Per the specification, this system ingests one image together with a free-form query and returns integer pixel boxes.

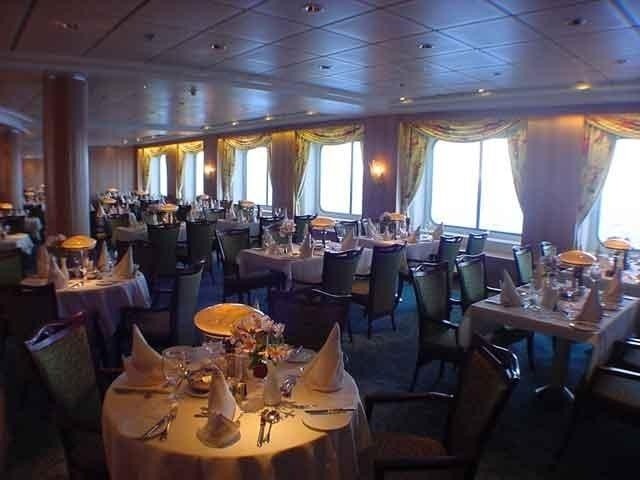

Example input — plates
[566,319,603,333]
[112,374,177,393]
[286,348,317,363]
[301,407,353,430]
[181,386,212,399]
[117,412,167,439]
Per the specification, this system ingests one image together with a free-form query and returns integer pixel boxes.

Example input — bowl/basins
[187,369,217,391]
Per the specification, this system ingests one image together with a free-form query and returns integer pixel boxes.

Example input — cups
[230,381,246,399]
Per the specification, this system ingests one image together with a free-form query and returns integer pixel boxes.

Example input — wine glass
[162,349,186,402]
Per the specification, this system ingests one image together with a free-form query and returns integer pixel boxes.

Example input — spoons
[263,410,281,444]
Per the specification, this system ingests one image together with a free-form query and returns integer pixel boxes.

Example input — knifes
[256,408,268,448]
[304,408,357,415]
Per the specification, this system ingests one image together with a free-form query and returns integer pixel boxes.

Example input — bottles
[263,360,281,406]
